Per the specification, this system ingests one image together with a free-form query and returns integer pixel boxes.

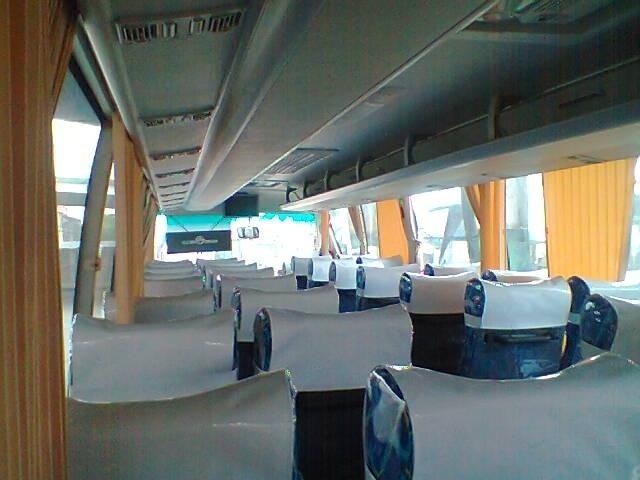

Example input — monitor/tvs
[225,193,259,217]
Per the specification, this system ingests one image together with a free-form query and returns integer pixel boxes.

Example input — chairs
[67,366,298,477]
[464,274,572,377]
[573,292,639,367]
[362,351,638,475]
[100,252,637,321]
[252,304,415,475]
[66,306,239,405]
